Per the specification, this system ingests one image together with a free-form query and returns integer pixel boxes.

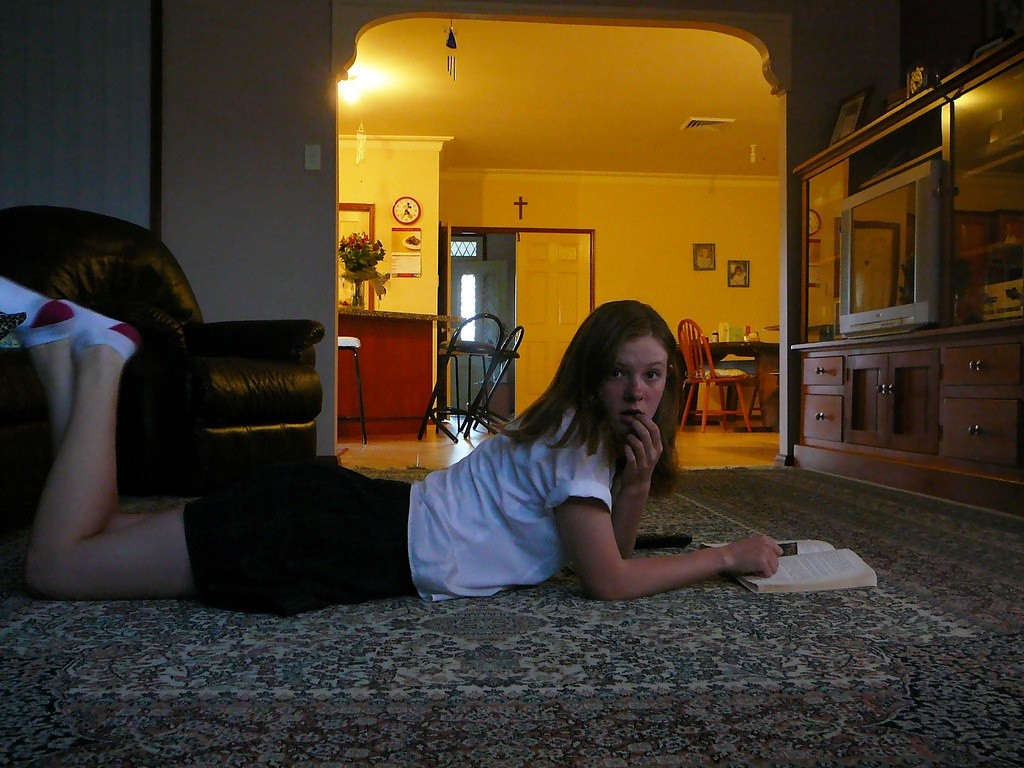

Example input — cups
[819,325,833,340]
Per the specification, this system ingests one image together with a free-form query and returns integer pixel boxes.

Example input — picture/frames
[827,85,871,147]
[728,260,749,287]
[692,243,716,271]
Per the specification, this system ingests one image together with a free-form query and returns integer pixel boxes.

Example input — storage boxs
[728,327,744,341]
[951,278,1024,326]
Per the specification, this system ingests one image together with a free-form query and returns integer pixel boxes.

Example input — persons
[1,275,786,611]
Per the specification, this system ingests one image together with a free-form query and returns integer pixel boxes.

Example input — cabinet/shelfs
[789,316,1024,470]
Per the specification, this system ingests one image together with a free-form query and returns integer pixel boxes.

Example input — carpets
[0,466,1024,768]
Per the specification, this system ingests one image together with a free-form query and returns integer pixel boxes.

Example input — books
[700,538,878,594]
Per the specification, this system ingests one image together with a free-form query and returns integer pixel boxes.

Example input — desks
[662,341,780,431]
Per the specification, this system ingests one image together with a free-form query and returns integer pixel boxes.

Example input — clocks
[809,209,822,236]
[392,196,421,224]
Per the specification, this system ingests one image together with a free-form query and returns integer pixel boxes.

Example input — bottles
[701,331,718,344]
[744,326,760,342]
[718,319,729,342]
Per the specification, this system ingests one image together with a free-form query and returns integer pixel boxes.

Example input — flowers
[339,231,389,311]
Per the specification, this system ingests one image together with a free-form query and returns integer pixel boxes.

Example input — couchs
[0,205,324,517]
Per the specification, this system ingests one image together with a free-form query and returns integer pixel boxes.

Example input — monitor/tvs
[839,158,945,339]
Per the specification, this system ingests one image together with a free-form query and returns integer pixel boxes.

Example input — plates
[402,238,420,250]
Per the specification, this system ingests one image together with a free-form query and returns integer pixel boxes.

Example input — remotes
[634,533,693,551]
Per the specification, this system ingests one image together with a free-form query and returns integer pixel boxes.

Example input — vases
[351,278,365,307]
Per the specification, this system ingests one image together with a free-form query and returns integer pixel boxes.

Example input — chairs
[677,320,754,433]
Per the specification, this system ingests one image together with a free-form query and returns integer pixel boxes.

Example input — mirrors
[338,203,375,311]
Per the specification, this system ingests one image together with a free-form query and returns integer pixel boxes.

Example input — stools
[416,313,525,445]
[338,336,368,445]
